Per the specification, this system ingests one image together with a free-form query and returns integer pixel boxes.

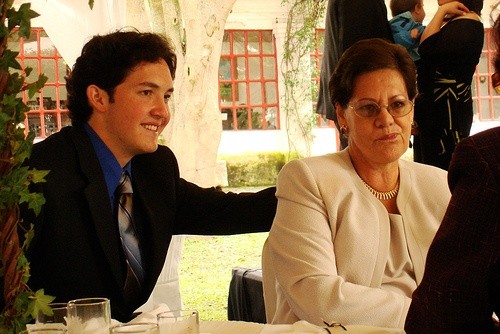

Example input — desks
[25,319,406,334]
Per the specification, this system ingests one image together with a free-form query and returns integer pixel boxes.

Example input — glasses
[341,99,418,118]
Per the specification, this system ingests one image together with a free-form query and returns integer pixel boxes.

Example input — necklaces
[361,178,400,200]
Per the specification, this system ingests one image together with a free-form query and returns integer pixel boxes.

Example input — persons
[16,26,278,322]
[404,14,500,334]
[316,0,396,151]
[388,0,484,171]
[268,39,452,329]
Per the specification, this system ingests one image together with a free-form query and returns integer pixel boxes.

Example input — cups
[109,322,160,334]
[156,309,200,334]
[67,298,111,334]
[35,302,68,334]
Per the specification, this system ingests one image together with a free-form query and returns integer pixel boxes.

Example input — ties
[116,173,144,310]
